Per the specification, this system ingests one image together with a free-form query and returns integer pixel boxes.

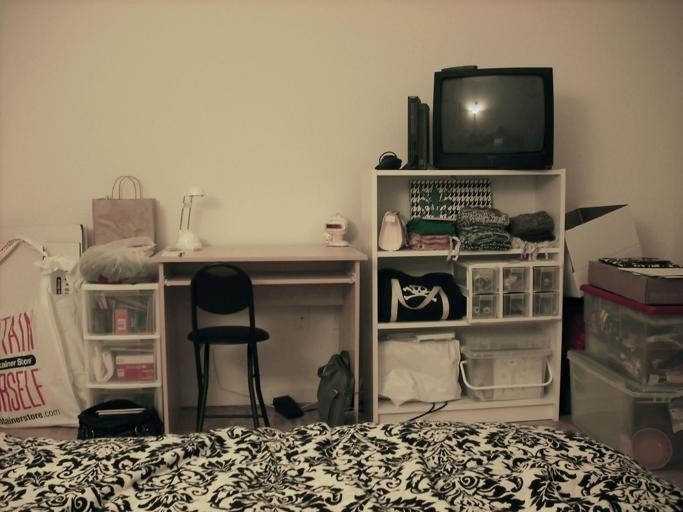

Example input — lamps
[174,187,206,251]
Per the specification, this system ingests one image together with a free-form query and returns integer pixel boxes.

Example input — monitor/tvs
[433,67,554,169]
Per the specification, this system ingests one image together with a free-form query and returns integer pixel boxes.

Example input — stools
[184,262,272,430]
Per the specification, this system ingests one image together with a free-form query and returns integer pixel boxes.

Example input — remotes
[441,65,477,72]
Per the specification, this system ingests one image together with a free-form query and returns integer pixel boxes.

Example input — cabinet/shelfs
[369,164,568,429]
[81,276,163,425]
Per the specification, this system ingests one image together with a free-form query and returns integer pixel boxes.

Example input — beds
[0,419,681,512]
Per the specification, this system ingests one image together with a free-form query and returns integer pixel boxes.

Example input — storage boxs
[457,324,552,351]
[566,346,683,469]
[564,204,644,300]
[451,259,561,319]
[580,281,683,387]
[459,344,555,400]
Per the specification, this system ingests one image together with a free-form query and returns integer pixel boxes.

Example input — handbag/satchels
[379,210,407,251]
[378,270,466,323]
[77,398,163,438]
[315,351,354,426]
[92,176,155,247]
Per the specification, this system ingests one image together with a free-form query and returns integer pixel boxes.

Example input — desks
[150,241,369,432]
